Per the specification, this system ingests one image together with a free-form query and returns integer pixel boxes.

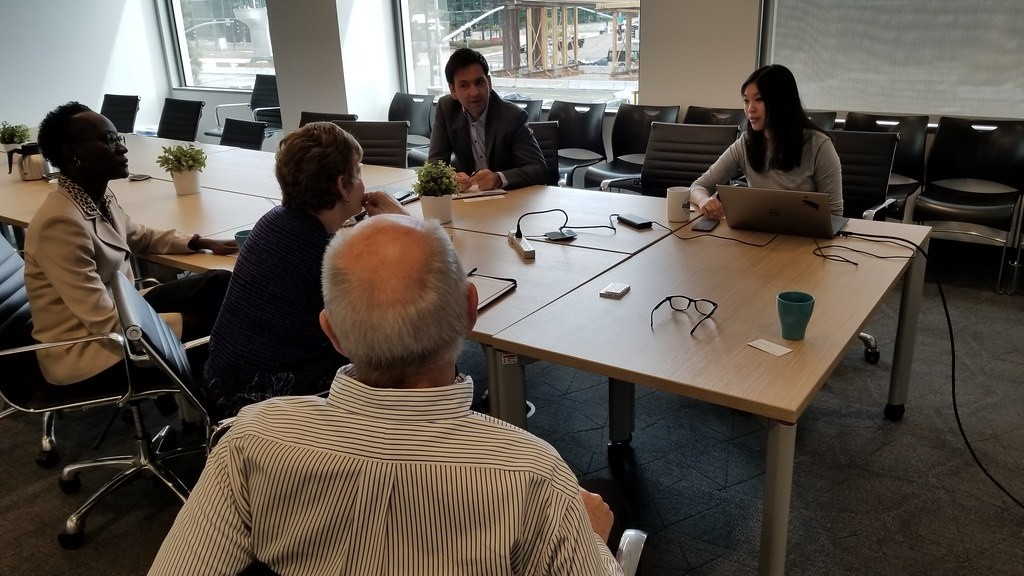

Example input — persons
[428,47,548,191]
[688,64,843,221]
[203,122,411,441]
[24,102,238,395]
[152,215,625,576]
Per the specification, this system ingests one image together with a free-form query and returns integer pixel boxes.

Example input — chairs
[100,75,1024,296]
[0,233,208,552]
[110,270,331,454]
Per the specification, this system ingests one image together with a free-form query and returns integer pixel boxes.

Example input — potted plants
[0,121,33,164]
[413,159,462,224]
[156,143,207,196]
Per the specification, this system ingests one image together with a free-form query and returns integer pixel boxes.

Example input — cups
[667,186,691,223]
[776,291,814,341]
[234,229,252,250]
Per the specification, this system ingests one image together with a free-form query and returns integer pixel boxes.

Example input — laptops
[716,184,850,239]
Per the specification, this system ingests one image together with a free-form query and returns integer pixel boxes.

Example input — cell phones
[692,217,720,232]
[393,191,410,200]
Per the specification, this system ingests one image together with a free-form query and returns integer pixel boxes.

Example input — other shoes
[180,428,204,447]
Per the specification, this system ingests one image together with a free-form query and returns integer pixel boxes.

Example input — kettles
[7,142,51,180]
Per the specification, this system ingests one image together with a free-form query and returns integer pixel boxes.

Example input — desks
[0,125,933,576]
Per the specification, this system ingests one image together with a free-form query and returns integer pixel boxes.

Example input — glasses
[651,295,717,335]
[80,132,125,149]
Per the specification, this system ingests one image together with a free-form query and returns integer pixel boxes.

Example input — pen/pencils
[468,167,482,179]
[466,267,478,277]
[397,193,410,201]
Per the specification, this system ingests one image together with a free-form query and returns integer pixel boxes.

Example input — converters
[616,212,652,229]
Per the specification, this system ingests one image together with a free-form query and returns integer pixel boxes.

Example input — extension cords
[508,230,535,258]
